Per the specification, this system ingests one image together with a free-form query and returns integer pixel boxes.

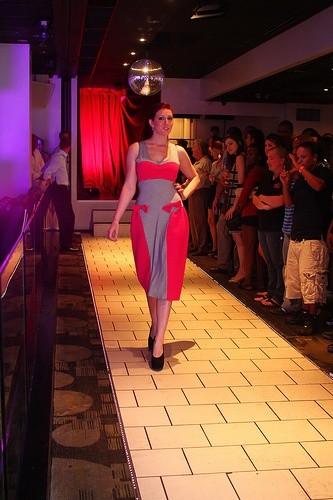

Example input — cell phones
[284,156,292,171]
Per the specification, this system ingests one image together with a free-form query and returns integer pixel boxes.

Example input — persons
[43,139,80,251]
[279,141,330,326]
[182,137,213,255]
[108,102,200,371]
[208,140,225,256]
[253,146,285,307]
[210,151,239,272]
[32,133,45,182]
[239,143,269,289]
[245,130,266,172]
[276,119,296,154]
[51,131,70,175]
[224,135,246,284]
[175,120,333,352]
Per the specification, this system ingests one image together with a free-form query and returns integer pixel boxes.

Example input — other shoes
[260,297,282,307]
[321,328,333,339]
[190,250,268,295]
[287,311,309,324]
[147,335,156,351]
[327,344,333,352]
[63,247,79,251]
[150,350,164,371]
[301,314,317,336]
[252,296,266,302]
[269,307,290,315]
